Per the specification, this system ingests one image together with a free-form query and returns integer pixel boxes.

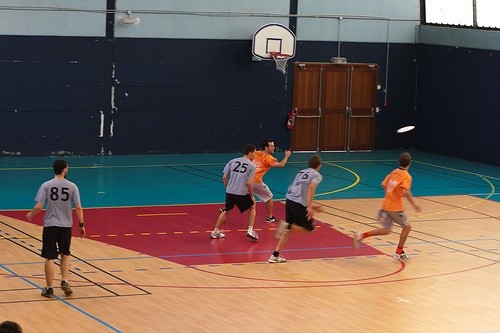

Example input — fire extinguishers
[286,106,298,129]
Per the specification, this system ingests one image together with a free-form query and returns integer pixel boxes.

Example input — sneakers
[41,287,53,298]
[61,280,73,296]
[268,254,286,262]
[266,216,279,222]
[210,231,225,239]
[393,252,410,261]
[247,230,258,239]
[354,232,362,248]
[276,221,287,238]
[220,208,225,213]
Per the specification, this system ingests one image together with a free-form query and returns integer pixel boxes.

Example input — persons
[210,145,259,240]
[268,156,322,263]
[26,160,85,298]
[0,320,22,333]
[352,151,420,261]
[250,140,291,223]
